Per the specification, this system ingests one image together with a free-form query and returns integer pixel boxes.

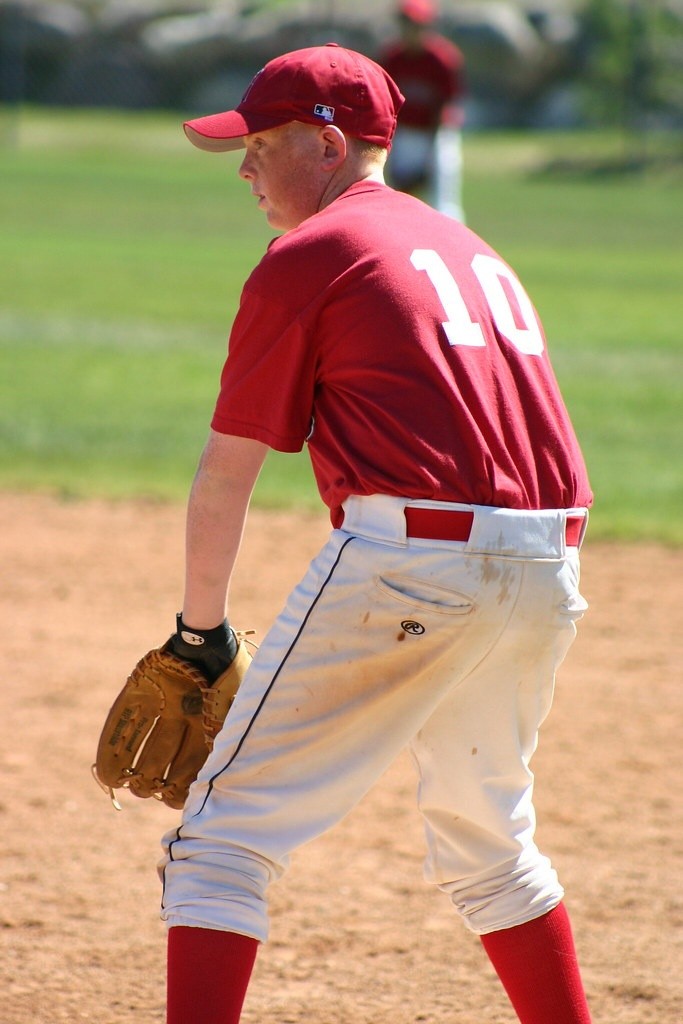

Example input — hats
[182,42,406,154]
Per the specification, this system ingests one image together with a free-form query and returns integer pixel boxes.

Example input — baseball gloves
[92,616,257,812]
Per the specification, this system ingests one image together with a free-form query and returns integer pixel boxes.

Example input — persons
[92,43,591,1024]
[368,0,467,220]
